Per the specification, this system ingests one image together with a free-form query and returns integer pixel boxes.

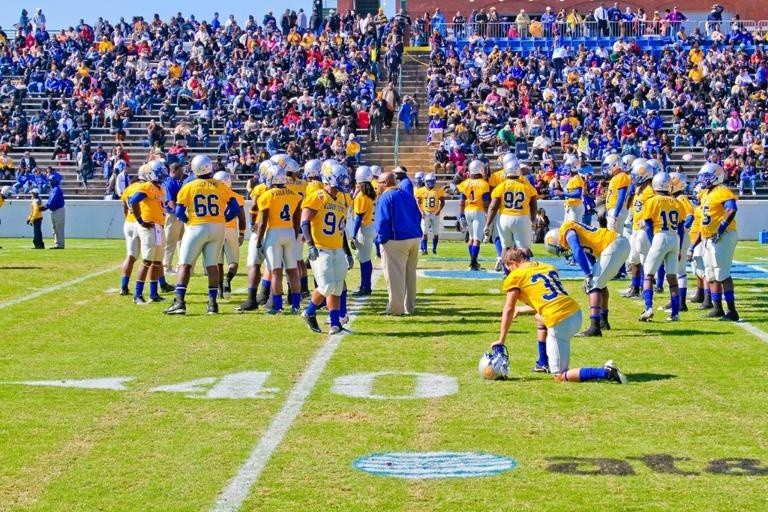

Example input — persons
[489,245,629,385]
[0,0,422,202]
[395,0,768,201]
[24,187,46,250]
[0,184,15,249]
[115,149,742,330]
[38,171,68,250]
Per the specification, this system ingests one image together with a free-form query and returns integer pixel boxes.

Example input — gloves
[612,216,617,228]
[459,217,467,228]
[712,231,721,244]
[239,224,357,270]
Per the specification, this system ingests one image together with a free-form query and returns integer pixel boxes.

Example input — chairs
[451,32,665,60]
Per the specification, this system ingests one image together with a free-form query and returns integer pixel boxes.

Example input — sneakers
[534,360,550,373]
[605,360,629,384]
[574,322,610,337]
[465,259,739,322]
[121,280,371,335]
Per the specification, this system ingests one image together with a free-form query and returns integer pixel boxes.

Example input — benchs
[661,108,708,180]
[8,91,247,199]
